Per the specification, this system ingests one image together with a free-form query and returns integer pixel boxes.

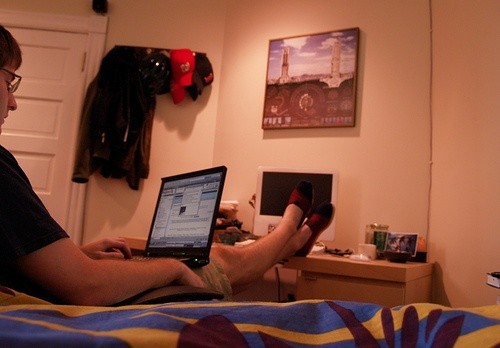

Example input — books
[486,272,500,289]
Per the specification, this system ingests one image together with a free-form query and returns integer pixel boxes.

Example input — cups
[358,244,376,260]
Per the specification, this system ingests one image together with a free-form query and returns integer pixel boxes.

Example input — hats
[169,48,195,86]
[187,53,214,95]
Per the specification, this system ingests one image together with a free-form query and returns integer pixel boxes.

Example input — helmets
[138,53,170,90]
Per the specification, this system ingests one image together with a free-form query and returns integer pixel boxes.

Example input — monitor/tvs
[253,169,338,242]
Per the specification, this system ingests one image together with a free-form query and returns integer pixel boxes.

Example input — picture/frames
[384,232,419,256]
[261,26,360,130]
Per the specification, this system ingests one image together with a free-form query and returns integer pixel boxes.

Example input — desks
[120,236,435,308]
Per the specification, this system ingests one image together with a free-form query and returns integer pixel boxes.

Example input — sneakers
[282,181,314,230]
[294,201,335,256]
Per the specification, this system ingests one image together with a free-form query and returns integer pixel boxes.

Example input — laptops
[129,165,228,270]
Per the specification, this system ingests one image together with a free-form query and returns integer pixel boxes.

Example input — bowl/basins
[384,251,411,263]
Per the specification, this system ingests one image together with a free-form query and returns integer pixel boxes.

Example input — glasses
[0,68,22,94]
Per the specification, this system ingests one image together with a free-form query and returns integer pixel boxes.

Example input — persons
[0,25,336,307]
